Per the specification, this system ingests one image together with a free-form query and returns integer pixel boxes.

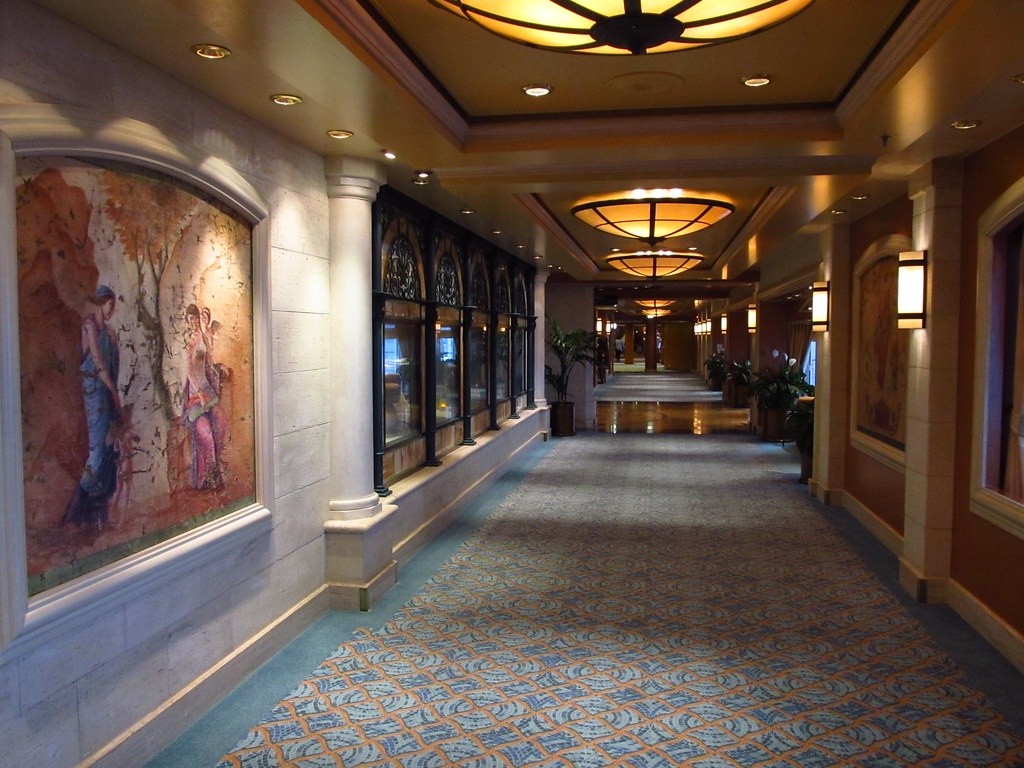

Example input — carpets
[144,433,1024,768]
[594,373,725,402]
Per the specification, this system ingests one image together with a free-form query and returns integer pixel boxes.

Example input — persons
[656,333,661,362]
[597,337,610,384]
[615,336,624,362]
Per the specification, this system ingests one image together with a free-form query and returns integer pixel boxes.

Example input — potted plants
[704,357,724,391]
[751,358,815,442]
[545,315,608,438]
[725,360,753,408]
[598,337,609,384]
[785,398,814,484]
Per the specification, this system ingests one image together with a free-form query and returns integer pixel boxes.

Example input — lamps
[435,320,441,334]
[721,313,727,334]
[634,300,677,308]
[694,323,698,335]
[426,0,816,56]
[812,282,828,332]
[897,251,924,329]
[571,197,736,247]
[707,319,712,335]
[641,309,671,318]
[698,322,702,335]
[605,321,610,335]
[605,254,704,278]
[702,320,706,335]
[610,322,617,330]
[596,318,602,334]
[748,304,756,333]
